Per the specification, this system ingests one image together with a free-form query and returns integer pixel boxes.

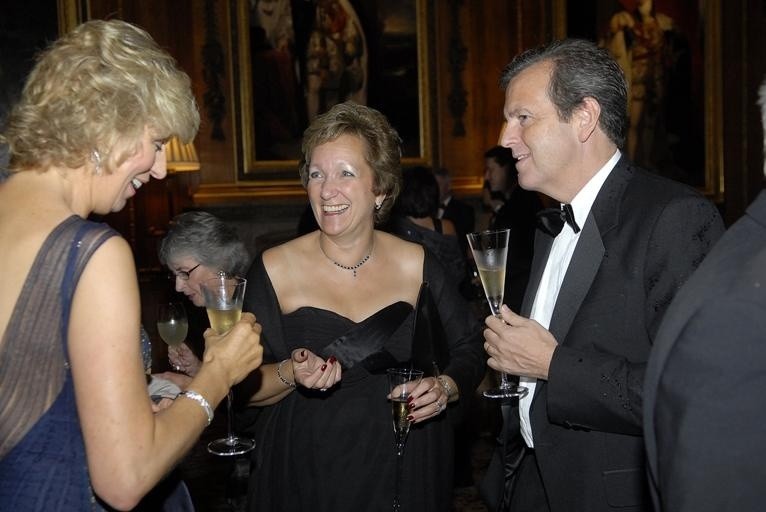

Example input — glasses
[167,264,200,280]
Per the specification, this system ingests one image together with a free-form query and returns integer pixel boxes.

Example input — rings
[435,400,441,409]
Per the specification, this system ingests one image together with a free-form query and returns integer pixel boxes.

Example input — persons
[0,18,265,512]
[143,209,257,393]
[401,146,545,315]
[235,96,477,512]
[480,36,726,512]
[640,78,765,512]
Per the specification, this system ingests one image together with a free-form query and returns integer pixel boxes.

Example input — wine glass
[154,298,190,377]
[464,227,530,398]
[197,275,257,457]
[382,366,426,511]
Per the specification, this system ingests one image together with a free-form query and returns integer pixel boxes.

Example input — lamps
[162,133,201,225]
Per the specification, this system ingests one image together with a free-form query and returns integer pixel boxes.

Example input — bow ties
[535,204,580,237]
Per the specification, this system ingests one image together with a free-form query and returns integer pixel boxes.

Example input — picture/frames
[226,1,439,190]
[0,0,95,180]
[551,1,727,207]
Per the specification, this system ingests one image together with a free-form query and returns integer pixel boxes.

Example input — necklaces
[321,232,373,280]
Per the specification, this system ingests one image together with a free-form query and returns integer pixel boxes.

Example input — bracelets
[276,358,297,389]
[182,391,214,423]
[434,376,453,400]
